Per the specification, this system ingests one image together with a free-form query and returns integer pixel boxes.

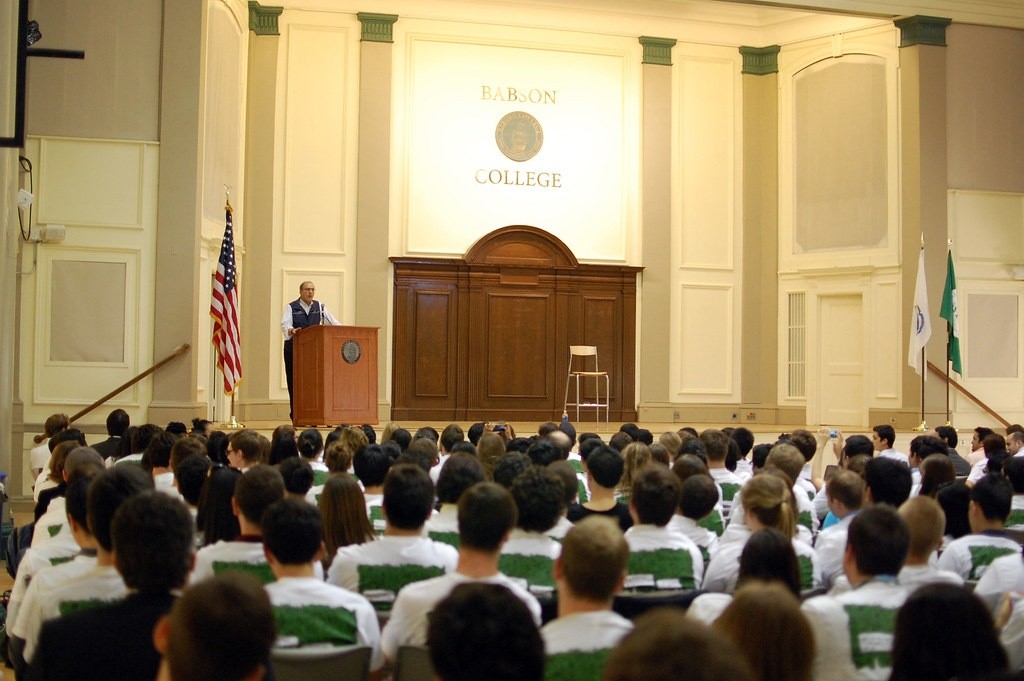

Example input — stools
[563,345,610,433]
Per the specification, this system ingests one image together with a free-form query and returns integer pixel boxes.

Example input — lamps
[39,224,66,244]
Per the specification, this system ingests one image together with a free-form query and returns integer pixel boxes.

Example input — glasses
[225,449,236,456]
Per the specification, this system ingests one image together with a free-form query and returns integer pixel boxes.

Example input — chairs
[393,644,434,681]
[271,645,372,681]
[613,588,709,621]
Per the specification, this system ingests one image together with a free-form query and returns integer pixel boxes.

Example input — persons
[6,409,1024,681]
[281,281,342,422]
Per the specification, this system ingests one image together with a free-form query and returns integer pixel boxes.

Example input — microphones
[321,304,325,317]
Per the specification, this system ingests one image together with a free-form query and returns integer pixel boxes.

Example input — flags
[210,202,244,396]
[939,250,963,377]
[907,242,931,381]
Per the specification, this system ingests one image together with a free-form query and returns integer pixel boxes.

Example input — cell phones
[494,424,507,432]
[830,430,839,438]
